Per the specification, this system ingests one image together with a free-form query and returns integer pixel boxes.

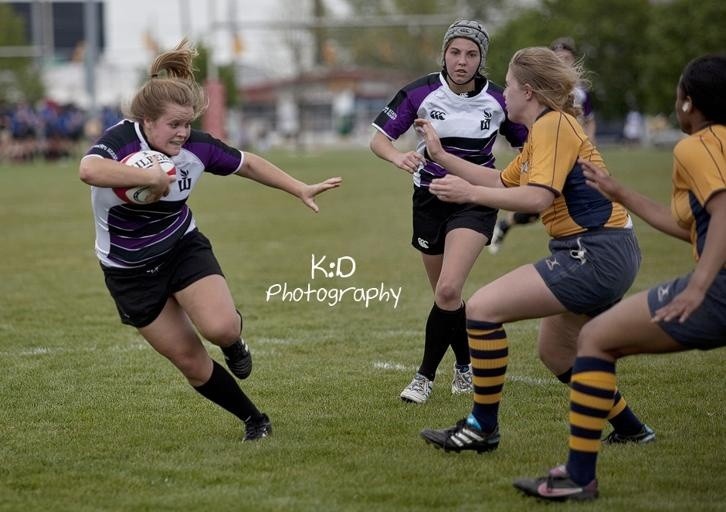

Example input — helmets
[442,17,491,63]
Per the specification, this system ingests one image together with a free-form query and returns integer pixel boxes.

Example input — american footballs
[111,151,176,204]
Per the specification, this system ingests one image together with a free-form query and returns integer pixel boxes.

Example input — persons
[1,94,129,166]
[367,16,530,406]
[77,35,344,444]
[411,44,658,455]
[509,45,726,503]
[484,35,600,261]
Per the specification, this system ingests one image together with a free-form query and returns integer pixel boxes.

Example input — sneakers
[221,337,252,380]
[450,360,472,395]
[601,424,656,446]
[399,373,434,404]
[241,412,272,443]
[512,464,599,502]
[420,418,500,455]
